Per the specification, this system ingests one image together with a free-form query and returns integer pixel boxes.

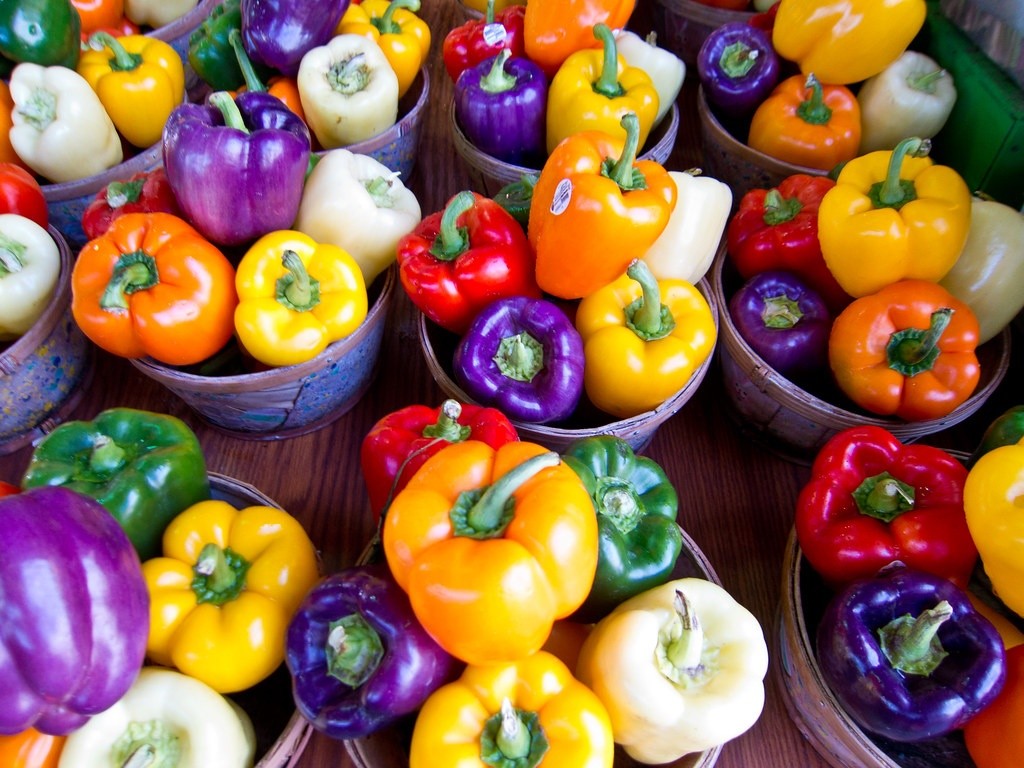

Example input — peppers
[1,1,1023,766]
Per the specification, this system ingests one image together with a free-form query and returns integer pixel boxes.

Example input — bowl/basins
[206,471,316,768]
[774,448,1024,768]
[143,1,223,100]
[1,222,90,457]
[130,260,396,441]
[706,235,1012,469]
[461,1,484,22]
[344,523,727,768]
[40,139,166,249]
[417,278,719,457]
[660,0,757,68]
[449,94,679,199]
[312,64,431,189]
[697,84,830,213]
